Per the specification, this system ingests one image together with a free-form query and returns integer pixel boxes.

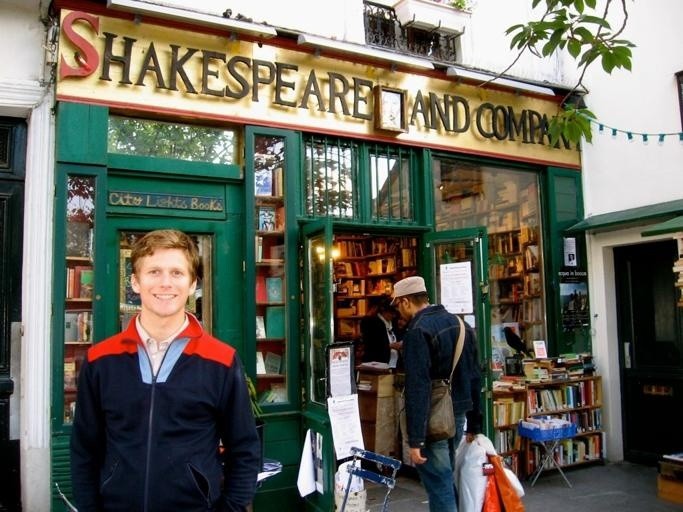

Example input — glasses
[387,328,395,342]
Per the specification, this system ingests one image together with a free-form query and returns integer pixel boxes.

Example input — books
[249,150,296,405]
[57,219,91,432]
[331,236,419,371]
[488,351,603,474]
[486,227,545,351]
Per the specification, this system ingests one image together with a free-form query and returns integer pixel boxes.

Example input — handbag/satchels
[451,433,526,512]
[398,378,457,447]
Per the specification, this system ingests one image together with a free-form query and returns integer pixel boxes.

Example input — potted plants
[391,0,472,34]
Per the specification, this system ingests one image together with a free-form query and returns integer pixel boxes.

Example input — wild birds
[502,326,534,360]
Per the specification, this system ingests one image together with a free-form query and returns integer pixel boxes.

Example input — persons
[67,229,262,512]
[387,274,485,511]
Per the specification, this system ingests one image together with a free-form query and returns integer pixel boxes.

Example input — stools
[527,435,575,489]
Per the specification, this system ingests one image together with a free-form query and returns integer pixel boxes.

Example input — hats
[389,275,427,307]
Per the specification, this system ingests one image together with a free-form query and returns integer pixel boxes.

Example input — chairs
[339,445,402,511]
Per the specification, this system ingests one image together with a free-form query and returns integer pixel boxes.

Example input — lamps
[295,30,435,79]
[446,68,555,98]
[105,0,277,39]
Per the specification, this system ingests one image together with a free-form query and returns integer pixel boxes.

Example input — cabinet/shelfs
[63,256,94,406]
[489,372,604,481]
[433,182,544,365]
[300,233,421,365]
[256,164,287,403]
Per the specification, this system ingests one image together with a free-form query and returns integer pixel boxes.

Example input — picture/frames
[117,248,197,315]
[374,85,410,135]
